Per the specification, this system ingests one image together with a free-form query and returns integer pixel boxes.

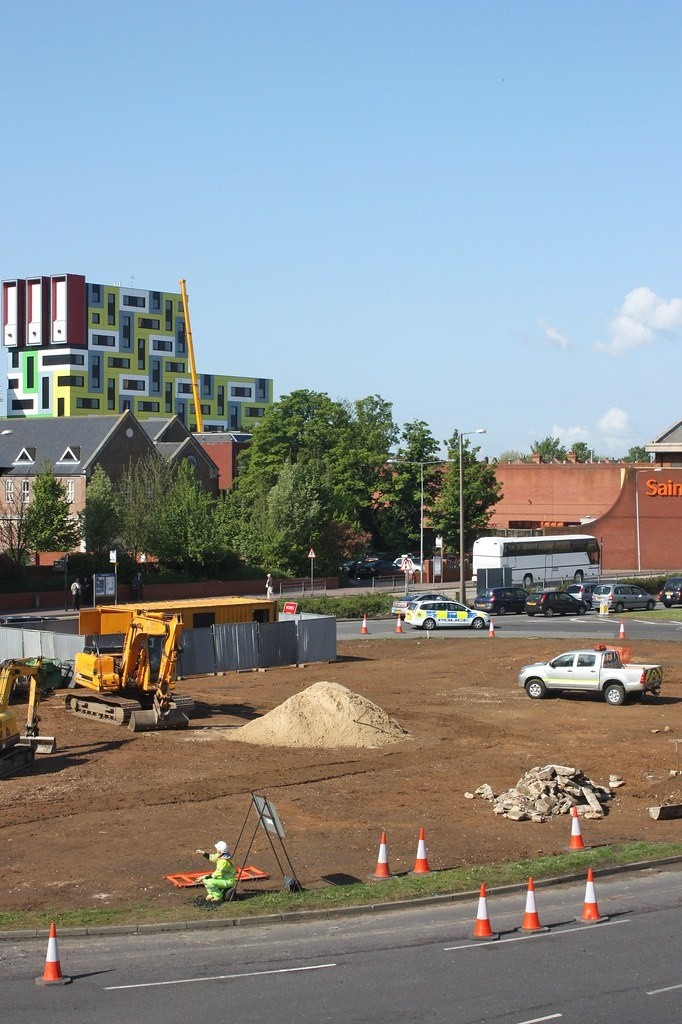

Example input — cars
[658,578,682,608]
[337,552,459,578]
[565,583,656,613]
[474,587,589,617]
[391,594,491,630]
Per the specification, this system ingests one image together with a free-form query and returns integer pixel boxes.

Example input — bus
[471,534,601,587]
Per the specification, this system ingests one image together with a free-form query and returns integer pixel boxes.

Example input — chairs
[580,655,595,665]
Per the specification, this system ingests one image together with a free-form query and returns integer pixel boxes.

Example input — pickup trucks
[518,649,663,706]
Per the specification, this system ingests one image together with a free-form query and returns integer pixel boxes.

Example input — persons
[265,574,274,599]
[196,841,236,903]
[131,572,144,602]
[71,576,91,611]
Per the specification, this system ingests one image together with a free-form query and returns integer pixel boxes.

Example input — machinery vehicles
[0,656,56,777]
[178,279,204,432]
[63,605,196,732]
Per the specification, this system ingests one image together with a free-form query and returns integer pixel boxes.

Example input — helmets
[215,841,229,853]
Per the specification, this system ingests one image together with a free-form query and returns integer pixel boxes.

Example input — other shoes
[211,898,223,902]
[205,895,214,900]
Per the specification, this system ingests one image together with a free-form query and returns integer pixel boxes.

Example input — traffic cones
[563,807,592,851]
[407,828,436,876]
[616,619,627,640]
[489,618,495,638]
[361,613,405,635]
[573,869,609,924]
[514,877,549,934]
[35,922,71,987]
[367,828,398,882]
[467,884,500,940]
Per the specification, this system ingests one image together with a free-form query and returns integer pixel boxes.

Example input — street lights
[636,468,662,572]
[387,429,486,604]
